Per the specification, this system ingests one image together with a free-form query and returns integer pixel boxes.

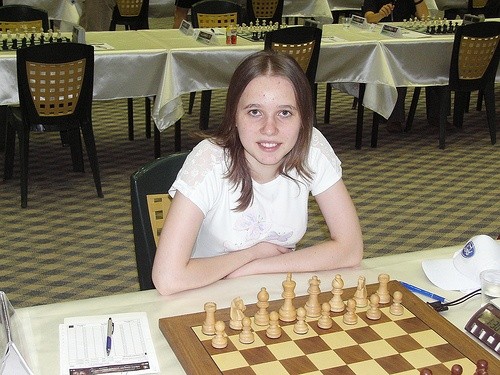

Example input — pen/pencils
[211,29,215,33]
[399,281,450,303]
[106,318,114,356]
[391,10,393,21]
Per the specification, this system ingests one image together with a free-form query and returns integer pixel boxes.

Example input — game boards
[398,25,456,36]
[235,26,288,41]
[157,279,500,375]
[0,36,72,55]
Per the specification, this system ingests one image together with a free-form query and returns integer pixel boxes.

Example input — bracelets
[414,0,424,5]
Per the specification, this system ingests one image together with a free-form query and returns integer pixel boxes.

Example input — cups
[341,18,351,29]
[480,268,500,309]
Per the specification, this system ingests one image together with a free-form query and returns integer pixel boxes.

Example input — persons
[360,0,456,132]
[152,50,363,296]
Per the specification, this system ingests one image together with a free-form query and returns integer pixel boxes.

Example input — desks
[136,24,378,152]
[326,18,500,148]
[0,239,500,375]
[0,30,169,161]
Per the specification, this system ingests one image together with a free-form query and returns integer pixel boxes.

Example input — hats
[421,235,500,292]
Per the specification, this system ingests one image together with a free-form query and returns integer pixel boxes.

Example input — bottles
[225,24,237,45]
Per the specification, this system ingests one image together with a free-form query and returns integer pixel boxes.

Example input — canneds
[225,25,237,45]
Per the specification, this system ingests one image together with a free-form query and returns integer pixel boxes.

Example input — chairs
[108,0,149,31]
[186,0,254,131]
[246,0,284,26]
[4,43,103,206]
[405,21,500,150]
[0,5,70,151]
[265,25,322,128]
